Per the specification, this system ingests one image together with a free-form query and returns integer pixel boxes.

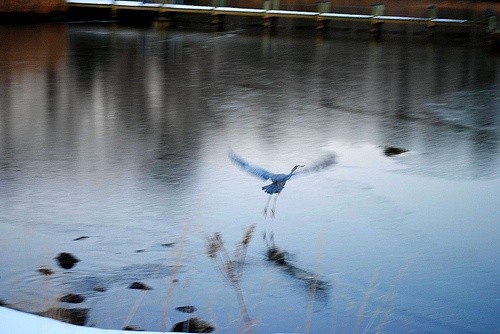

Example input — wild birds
[227,149,337,220]
